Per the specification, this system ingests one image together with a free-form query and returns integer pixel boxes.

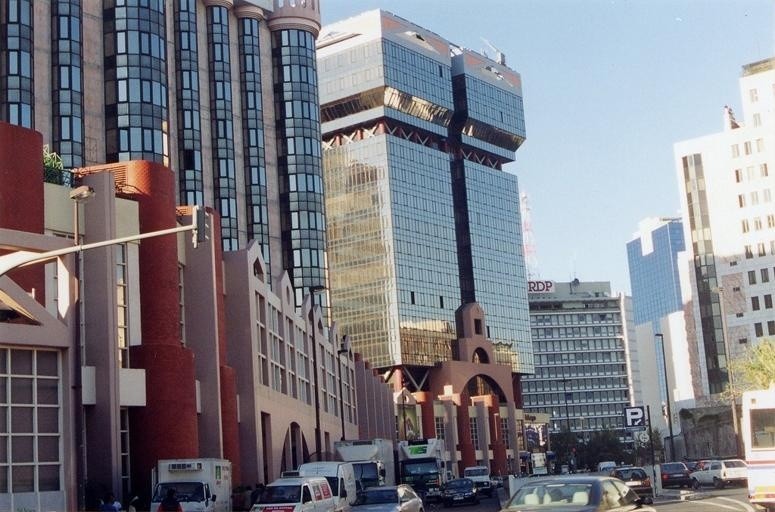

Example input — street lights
[655,333,678,464]
[557,378,574,435]
[711,285,743,461]
[307,281,333,463]
[338,348,348,443]
[67,184,97,510]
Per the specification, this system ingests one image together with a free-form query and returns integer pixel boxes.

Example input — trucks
[248,436,451,512]
[147,456,235,512]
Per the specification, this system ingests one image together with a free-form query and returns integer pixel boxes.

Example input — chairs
[572,492,589,504]
[755,430,773,447]
[523,494,539,505]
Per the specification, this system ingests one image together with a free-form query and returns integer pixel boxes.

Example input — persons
[233,483,264,510]
[95,488,184,512]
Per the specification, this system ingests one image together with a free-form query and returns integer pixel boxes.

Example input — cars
[490,474,505,487]
[502,476,665,512]
[440,474,482,506]
[557,457,754,507]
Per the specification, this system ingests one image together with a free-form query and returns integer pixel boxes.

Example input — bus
[740,390,775,512]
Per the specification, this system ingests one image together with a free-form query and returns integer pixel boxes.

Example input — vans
[463,466,497,497]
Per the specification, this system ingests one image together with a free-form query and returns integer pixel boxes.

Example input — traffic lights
[204,211,211,243]
